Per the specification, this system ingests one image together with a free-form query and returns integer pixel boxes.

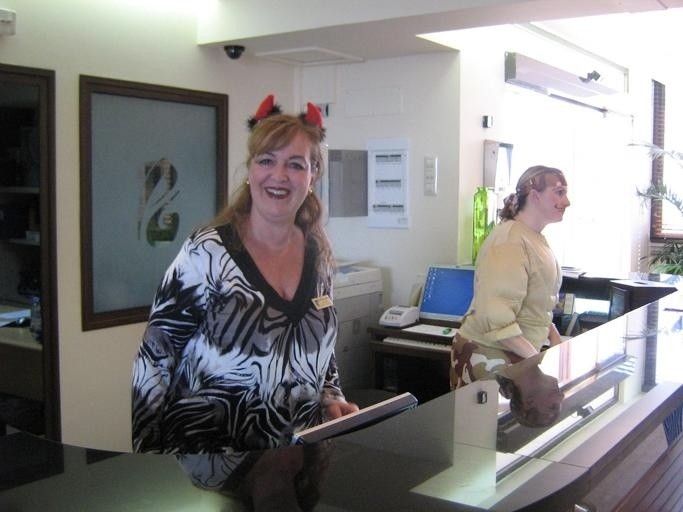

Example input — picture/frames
[78,71,229,331]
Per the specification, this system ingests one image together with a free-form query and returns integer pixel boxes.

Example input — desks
[365,317,461,390]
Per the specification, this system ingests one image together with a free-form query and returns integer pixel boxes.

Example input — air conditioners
[504,52,619,114]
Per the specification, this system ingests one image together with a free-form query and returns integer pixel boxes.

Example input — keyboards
[382,336,452,352]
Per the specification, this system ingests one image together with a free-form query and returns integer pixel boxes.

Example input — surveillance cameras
[224,45,245,60]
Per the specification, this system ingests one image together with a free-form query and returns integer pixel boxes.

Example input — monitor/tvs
[607,280,677,319]
[417,264,475,322]
[288,391,419,444]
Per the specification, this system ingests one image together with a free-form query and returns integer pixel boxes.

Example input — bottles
[30,297,42,335]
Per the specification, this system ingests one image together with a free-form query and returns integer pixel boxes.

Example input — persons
[174,441,335,512]
[497,352,565,429]
[132,116,360,452]
[450,167,572,391]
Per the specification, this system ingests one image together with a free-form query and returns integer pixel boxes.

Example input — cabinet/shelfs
[0,187,44,405]
